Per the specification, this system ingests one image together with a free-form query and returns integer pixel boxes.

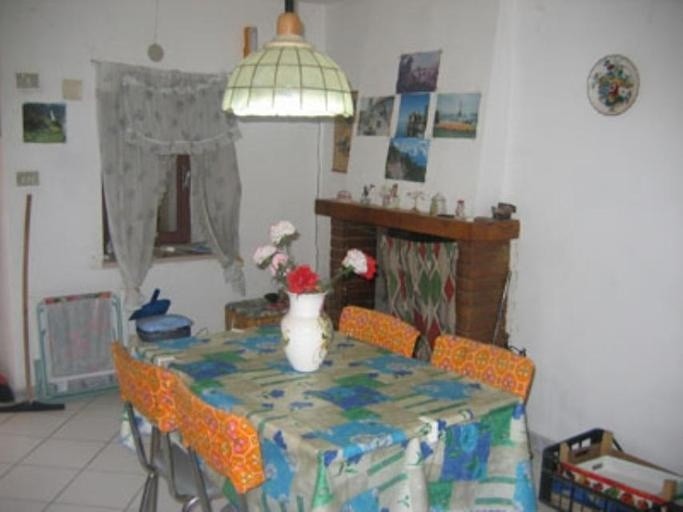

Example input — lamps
[217,0,361,122]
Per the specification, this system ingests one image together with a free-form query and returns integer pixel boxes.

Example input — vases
[276,283,334,376]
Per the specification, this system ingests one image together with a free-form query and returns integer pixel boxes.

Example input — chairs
[109,306,535,512]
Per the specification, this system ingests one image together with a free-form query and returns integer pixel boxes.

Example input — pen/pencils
[364,183,374,198]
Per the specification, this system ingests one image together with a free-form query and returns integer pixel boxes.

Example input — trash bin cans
[136,315,192,341]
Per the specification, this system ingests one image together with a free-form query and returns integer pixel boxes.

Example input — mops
[0,195,66,412]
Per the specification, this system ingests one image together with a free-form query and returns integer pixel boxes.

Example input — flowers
[250,218,375,301]
